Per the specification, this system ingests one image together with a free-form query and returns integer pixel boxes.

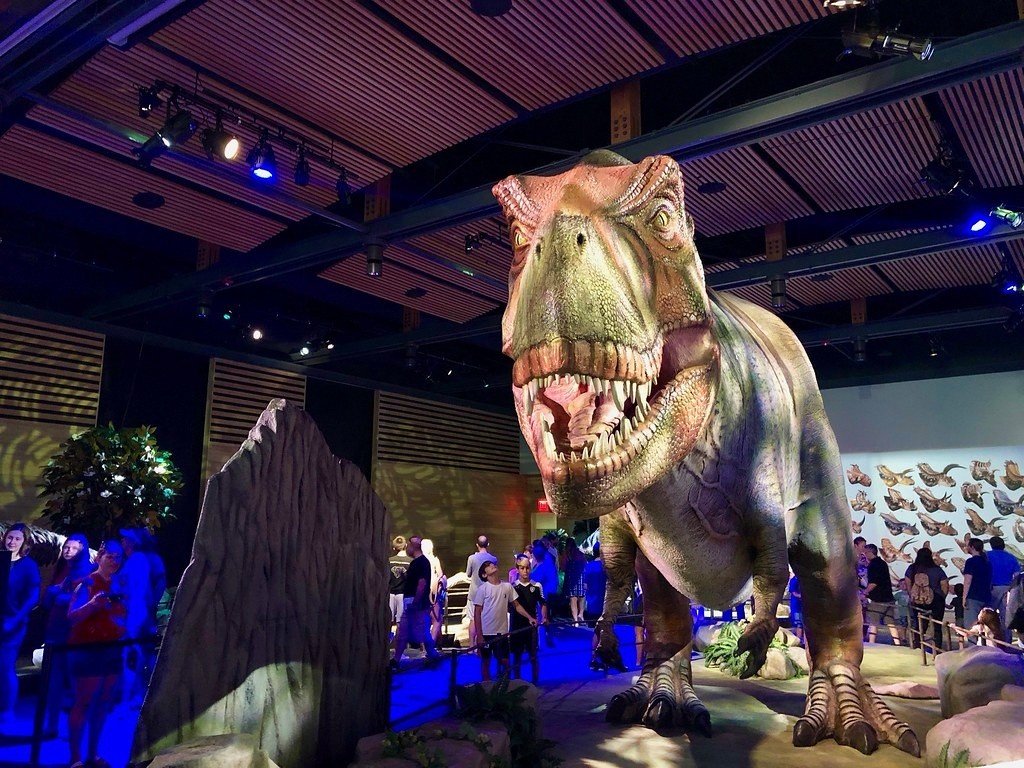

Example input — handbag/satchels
[437,574,448,602]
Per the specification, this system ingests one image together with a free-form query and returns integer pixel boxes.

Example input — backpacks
[912,573,934,605]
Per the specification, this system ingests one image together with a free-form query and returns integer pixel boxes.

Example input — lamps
[137,78,358,204]
[839,2,935,64]
[919,151,1024,239]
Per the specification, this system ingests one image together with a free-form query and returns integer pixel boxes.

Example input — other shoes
[390,658,398,671]
[84,757,110,768]
[41,730,58,740]
[572,614,586,627]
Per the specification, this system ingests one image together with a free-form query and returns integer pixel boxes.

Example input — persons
[853,536,1024,646]
[0,523,168,768]
[390,534,607,684]
[788,576,806,643]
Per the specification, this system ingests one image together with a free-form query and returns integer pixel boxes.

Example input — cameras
[104,593,121,603]
